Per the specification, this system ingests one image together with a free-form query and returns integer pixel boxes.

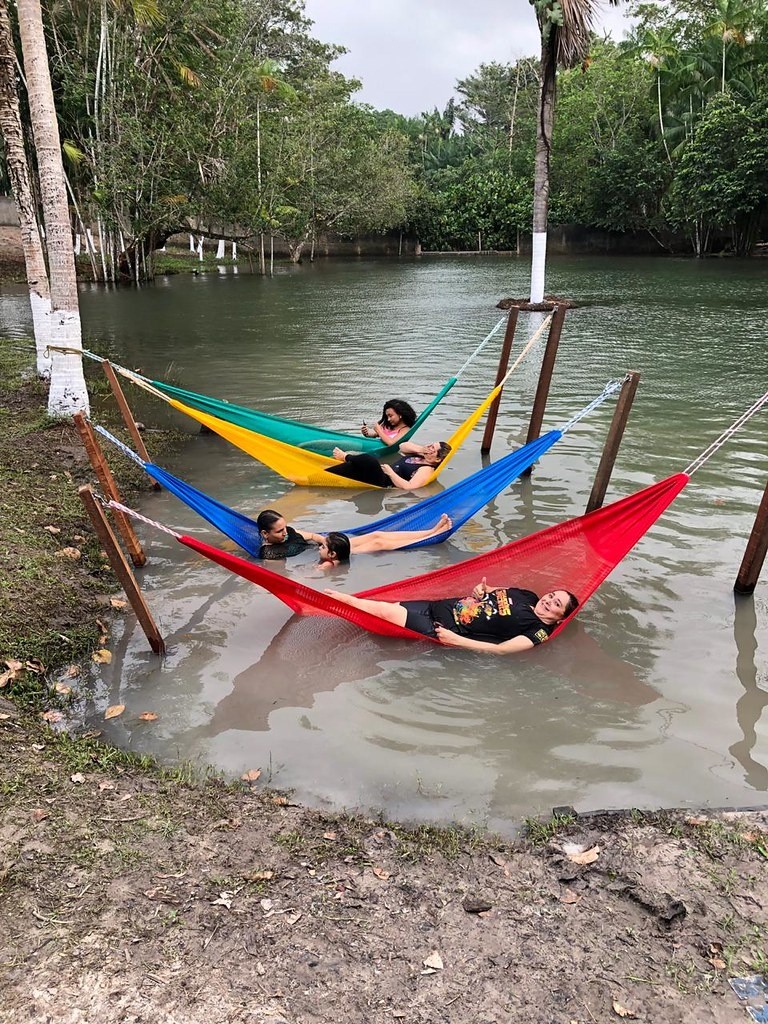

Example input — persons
[324,577,579,656]
[290,399,417,450]
[315,532,350,570]
[256,509,452,560]
[324,442,451,490]
